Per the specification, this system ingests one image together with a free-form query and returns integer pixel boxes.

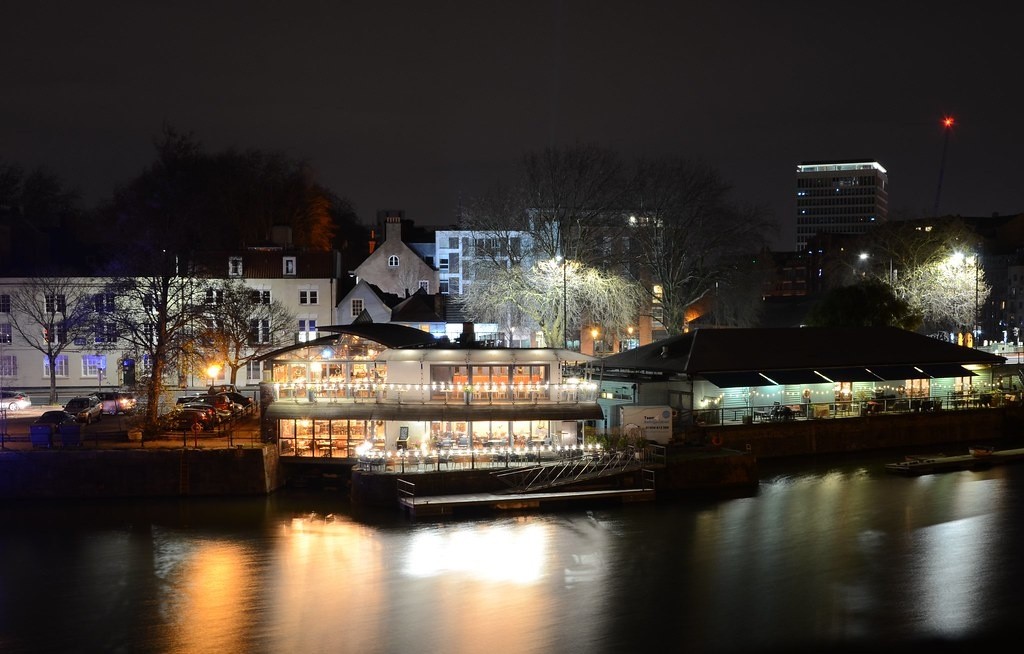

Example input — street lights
[555,254,567,350]
[594,330,605,358]
[628,327,633,350]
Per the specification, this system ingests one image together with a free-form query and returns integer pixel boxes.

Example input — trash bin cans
[60,425,81,449]
[29,425,53,450]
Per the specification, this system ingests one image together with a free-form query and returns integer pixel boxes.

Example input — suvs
[62,394,104,425]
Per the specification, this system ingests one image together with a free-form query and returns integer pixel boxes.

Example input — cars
[0,391,33,412]
[32,410,78,432]
[157,391,254,432]
[88,392,136,416]
